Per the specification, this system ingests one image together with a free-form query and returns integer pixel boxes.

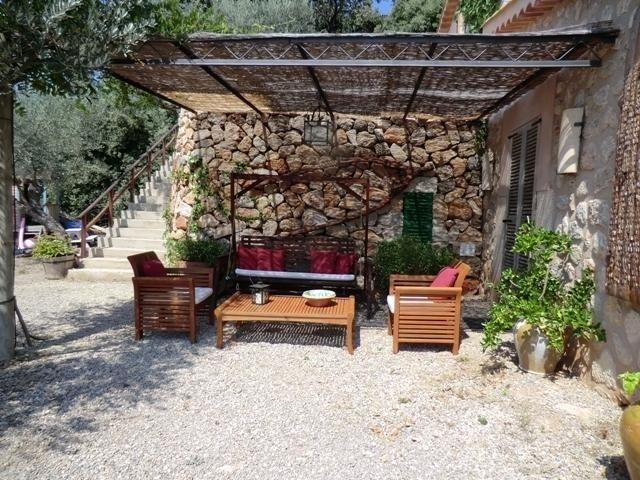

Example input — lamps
[481,152,494,191]
[556,106,585,175]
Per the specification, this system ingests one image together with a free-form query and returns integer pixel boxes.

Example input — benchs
[24,225,44,238]
[229,235,356,288]
[85,235,98,240]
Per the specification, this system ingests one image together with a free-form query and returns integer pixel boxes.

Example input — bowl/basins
[302,289,336,306]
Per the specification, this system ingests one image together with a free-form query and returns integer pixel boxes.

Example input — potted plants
[480,220,608,376]
[31,233,80,280]
[616,370,640,480]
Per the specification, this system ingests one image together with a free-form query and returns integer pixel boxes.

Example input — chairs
[127,251,215,343]
[387,258,471,355]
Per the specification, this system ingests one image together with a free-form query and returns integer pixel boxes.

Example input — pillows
[428,267,459,300]
[140,259,167,298]
[237,242,358,274]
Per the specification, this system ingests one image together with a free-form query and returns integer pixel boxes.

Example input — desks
[65,228,81,239]
